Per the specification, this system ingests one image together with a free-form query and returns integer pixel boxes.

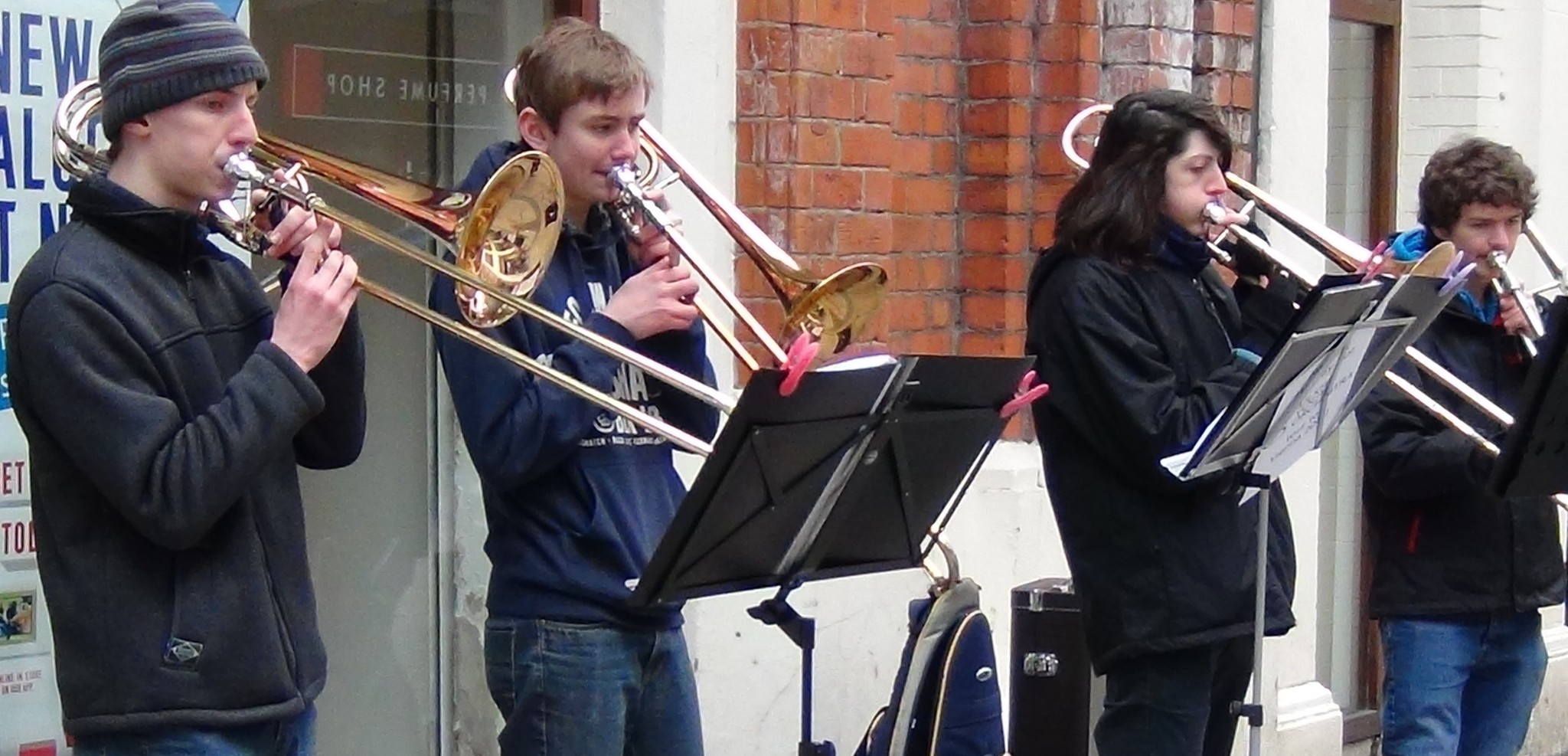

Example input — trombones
[1060,105,1515,457]
[50,77,740,459]
[506,65,887,372]
[1487,224,1568,363]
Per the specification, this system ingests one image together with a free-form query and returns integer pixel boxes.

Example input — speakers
[1009,577,1116,756]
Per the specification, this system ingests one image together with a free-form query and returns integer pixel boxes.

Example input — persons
[427,17,721,756]
[1354,138,1568,756]
[1023,86,1299,756]
[5,0,366,756]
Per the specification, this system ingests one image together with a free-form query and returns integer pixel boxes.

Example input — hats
[97,0,270,141]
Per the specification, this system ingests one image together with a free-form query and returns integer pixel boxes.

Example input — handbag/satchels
[847,530,1005,756]
